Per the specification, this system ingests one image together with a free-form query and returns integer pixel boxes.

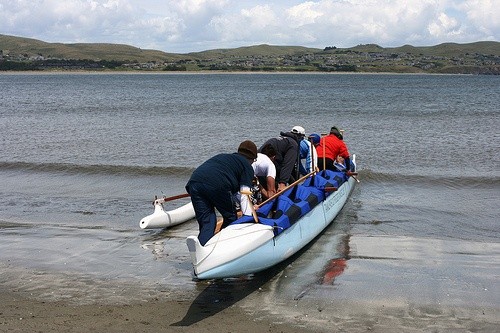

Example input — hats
[308,134,320,146]
[330,127,343,140]
[291,126,306,137]
[238,141,257,158]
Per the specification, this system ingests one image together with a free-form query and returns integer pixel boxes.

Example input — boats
[185,153,357,280]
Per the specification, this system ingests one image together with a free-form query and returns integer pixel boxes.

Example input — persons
[233,143,276,212]
[257,125,306,202]
[185,140,258,246]
[315,126,351,175]
[299,133,321,180]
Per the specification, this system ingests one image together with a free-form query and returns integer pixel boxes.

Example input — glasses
[253,158,257,162]
